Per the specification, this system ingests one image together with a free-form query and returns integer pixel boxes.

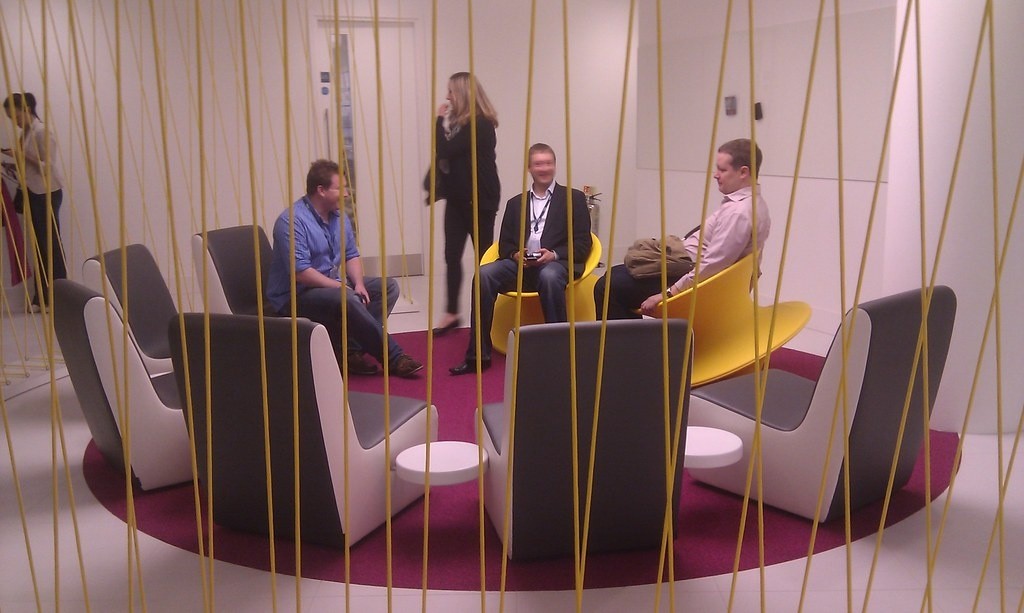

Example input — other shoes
[433,319,459,335]
[29,304,49,313]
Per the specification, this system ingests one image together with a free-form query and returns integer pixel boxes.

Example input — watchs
[666,288,673,298]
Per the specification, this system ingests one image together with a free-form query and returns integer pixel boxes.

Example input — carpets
[82,327,965,592]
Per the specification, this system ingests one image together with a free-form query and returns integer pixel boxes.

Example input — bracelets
[511,251,517,261]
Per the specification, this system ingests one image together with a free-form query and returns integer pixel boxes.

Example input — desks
[395,441,489,488]
[683,425,744,470]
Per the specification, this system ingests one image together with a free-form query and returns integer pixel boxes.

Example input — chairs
[82,243,173,374]
[474,317,694,565]
[633,248,813,389]
[687,285,957,528]
[167,311,440,553]
[53,277,201,493]
[479,231,603,355]
[191,224,272,314]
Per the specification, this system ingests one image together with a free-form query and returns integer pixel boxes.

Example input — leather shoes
[450,358,488,375]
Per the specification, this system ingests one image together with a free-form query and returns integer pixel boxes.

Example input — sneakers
[390,354,423,376]
[340,352,382,375]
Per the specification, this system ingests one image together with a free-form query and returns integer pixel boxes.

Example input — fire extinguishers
[586,192,602,237]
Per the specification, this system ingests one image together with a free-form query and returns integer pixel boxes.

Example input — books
[2,164,20,184]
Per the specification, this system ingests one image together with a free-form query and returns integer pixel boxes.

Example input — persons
[449,143,592,375]
[594,137,771,318]
[424,73,501,335]
[265,159,424,375]
[0,92,67,314]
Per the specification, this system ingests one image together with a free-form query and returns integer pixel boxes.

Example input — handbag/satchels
[624,234,695,280]
[13,187,24,214]
